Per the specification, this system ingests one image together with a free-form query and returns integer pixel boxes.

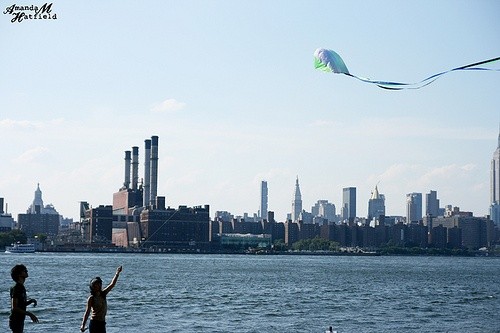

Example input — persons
[9,265,39,332]
[80,264,122,333]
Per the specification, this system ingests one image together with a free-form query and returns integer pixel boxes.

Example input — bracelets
[115,273,119,276]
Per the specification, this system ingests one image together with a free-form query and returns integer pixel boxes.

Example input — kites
[314,49,500,91]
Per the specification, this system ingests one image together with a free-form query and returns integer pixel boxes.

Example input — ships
[4,244,36,254]
